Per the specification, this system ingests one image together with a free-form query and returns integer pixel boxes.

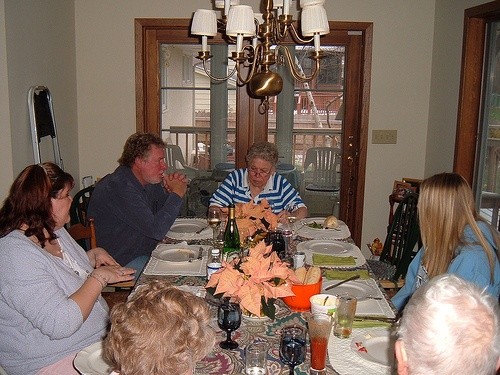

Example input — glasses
[247,165,273,177]
[394,318,407,361]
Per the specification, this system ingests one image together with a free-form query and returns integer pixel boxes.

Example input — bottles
[223,204,240,257]
[207,249,222,281]
[282,230,294,270]
[293,252,305,268]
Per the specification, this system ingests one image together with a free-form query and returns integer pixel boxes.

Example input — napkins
[312,254,356,265]
[352,315,392,328]
[327,270,369,280]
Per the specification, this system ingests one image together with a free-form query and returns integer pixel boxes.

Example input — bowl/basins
[281,278,322,313]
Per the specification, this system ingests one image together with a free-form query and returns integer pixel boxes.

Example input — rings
[119,270,125,275]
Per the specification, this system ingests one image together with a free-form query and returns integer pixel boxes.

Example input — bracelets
[89,273,107,288]
[295,212,301,220]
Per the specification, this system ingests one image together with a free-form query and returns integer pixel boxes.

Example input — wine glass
[278,324,307,375]
[217,303,242,349]
[208,206,223,248]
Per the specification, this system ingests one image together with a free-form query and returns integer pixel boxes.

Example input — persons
[208,141,308,224]
[394,273,500,375]
[389,172,500,316]
[0,160,137,375]
[103,279,217,375]
[86,131,188,281]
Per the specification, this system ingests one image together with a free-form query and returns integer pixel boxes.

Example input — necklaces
[252,188,261,191]
[21,227,62,255]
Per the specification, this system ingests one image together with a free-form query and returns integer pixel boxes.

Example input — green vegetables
[309,221,321,229]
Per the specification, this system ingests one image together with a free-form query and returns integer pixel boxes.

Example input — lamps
[191,0,331,115]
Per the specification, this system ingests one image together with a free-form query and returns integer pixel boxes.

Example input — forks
[188,253,194,262]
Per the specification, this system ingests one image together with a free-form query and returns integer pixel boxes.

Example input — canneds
[293,252,306,269]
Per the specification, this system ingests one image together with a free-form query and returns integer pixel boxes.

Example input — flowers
[233,196,278,242]
[205,240,294,327]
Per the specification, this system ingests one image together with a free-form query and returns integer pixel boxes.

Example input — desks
[124,219,399,375]
[213,161,298,194]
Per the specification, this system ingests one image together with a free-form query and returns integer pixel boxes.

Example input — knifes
[325,275,360,290]
[198,246,203,260]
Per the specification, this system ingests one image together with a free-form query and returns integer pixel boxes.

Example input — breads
[294,266,321,285]
[324,216,337,227]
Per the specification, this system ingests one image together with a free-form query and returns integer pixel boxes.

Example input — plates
[325,281,377,303]
[305,240,352,256]
[152,244,206,265]
[169,220,207,234]
[350,331,393,368]
[307,219,338,230]
[242,303,280,321]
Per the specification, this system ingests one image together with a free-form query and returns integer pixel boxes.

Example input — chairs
[364,191,422,288]
[301,147,344,210]
[163,144,201,186]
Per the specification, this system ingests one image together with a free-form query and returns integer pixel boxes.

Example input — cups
[245,343,267,375]
[266,228,285,258]
[310,293,338,320]
[333,294,357,338]
[306,313,334,372]
